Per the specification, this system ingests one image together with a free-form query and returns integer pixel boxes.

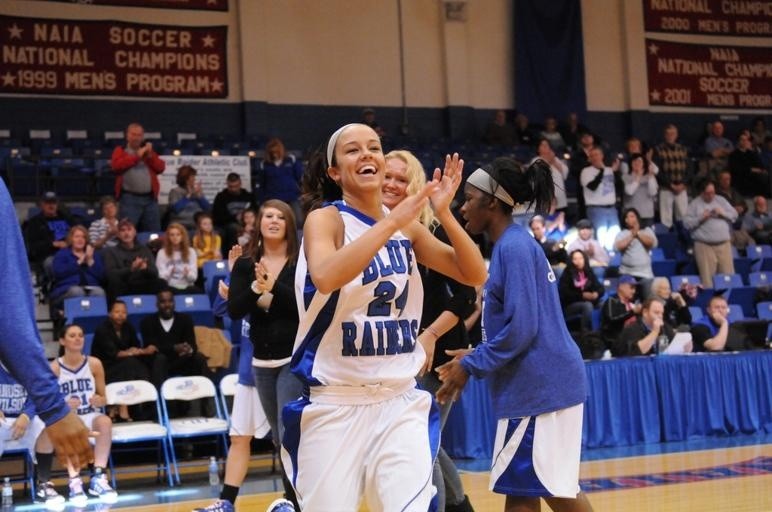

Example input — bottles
[207,454,220,485]
[1,477,14,512]
[658,334,670,355]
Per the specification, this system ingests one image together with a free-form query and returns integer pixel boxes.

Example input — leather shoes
[111,415,132,423]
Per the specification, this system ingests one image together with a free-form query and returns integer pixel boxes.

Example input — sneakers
[191,498,235,512]
[88,475,118,500]
[266,499,296,512]
[67,477,88,507]
[35,481,65,504]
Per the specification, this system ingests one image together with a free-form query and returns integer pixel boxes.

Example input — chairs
[161,376,231,484]
[7,141,234,354]
[104,380,175,488]
[221,373,242,435]
[519,208,770,351]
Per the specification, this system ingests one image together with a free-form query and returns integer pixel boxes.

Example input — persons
[21,122,222,424]
[2,180,97,469]
[200,110,596,511]
[1,324,119,505]
[572,122,772,359]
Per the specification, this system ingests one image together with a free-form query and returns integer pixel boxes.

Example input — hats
[576,219,591,227]
[41,191,58,201]
[617,275,638,284]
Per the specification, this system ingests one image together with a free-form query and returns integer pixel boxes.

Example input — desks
[458,351,772,463]
